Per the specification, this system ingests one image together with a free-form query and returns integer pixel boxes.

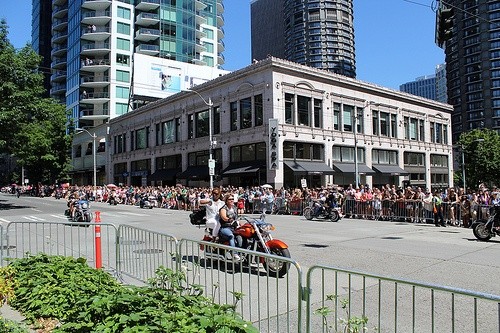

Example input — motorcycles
[199,207,291,278]
[471,203,500,242]
[65,203,91,228]
[303,201,342,222]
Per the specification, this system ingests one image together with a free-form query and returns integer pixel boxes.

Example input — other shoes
[234,254,240,260]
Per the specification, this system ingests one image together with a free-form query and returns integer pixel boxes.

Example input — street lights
[180,89,216,189]
[354,103,381,189]
[461,139,485,195]
[75,128,97,191]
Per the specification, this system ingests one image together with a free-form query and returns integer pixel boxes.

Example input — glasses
[228,198,234,200]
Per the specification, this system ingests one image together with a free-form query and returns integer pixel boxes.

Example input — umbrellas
[60,183,68,188]
[106,184,117,190]
[260,184,273,191]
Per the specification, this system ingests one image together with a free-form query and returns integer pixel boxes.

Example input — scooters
[109,195,119,205]
[139,195,156,209]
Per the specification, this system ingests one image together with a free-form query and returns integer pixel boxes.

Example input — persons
[199,187,225,252]
[218,194,248,264]
[1,183,500,235]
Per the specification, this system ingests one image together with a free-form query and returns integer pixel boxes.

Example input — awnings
[333,163,376,175]
[283,161,334,175]
[372,165,407,176]
[221,160,264,174]
[177,165,210,181]
[148,167,182,181]
